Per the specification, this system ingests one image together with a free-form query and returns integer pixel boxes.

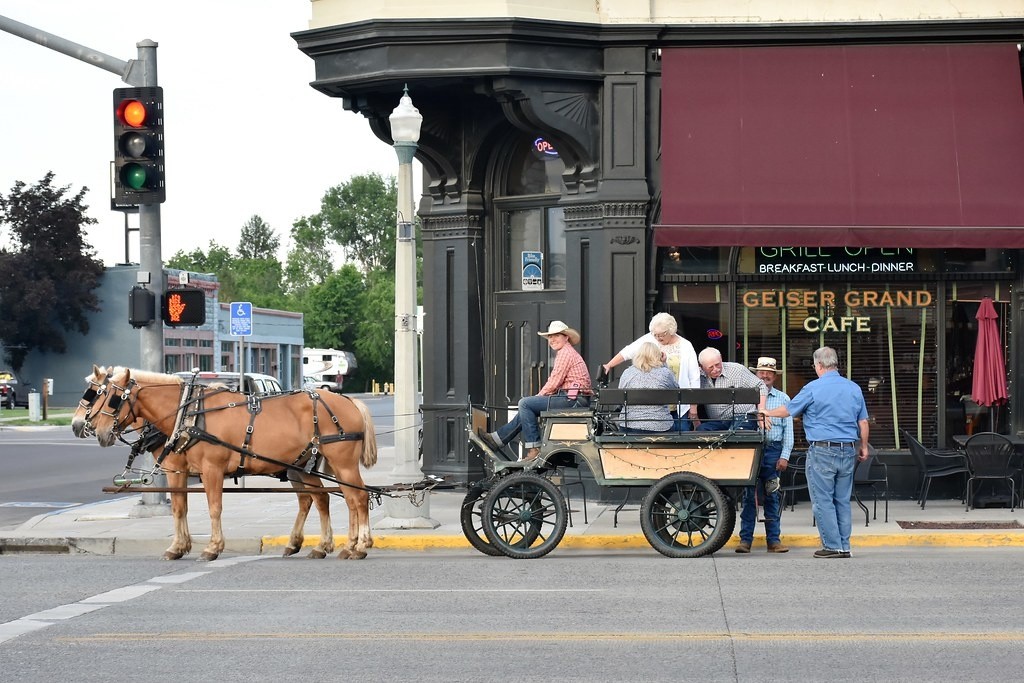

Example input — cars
[0,371,33,411]
[303,376,340,392]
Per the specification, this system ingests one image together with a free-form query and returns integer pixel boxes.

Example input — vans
[169,371,285,398]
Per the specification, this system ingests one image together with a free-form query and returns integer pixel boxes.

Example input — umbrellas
[971,295,1009,440]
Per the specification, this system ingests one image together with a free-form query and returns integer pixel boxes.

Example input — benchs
[539,385,766,444]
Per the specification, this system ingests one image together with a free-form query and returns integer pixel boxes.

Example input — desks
[800,454,885,527]
[952,434,1024,509]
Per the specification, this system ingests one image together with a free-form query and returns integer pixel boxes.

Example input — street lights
[386,82,423,476]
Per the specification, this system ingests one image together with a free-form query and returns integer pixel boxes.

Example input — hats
[537,320,581,345]
[748,356,784,375]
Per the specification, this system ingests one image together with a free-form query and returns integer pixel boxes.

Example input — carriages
[68,365,770,561]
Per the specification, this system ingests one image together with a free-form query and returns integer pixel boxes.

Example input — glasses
[756,361,776,367]
[654,331,668,338]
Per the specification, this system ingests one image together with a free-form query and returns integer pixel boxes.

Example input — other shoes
[736,540,750,553]
[478,427,499,452]
[814,546,850,558]
[767,542,789,552]
[520,449,540,463]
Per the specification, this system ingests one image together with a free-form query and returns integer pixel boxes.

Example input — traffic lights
[162,288,206,327]
[112,86,167,205]
[127,285,156,330]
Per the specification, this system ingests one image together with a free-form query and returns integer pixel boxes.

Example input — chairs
[777,452,816,527]
[963,432,1023,513]
[854,438,889,522]
[899,428,969,511]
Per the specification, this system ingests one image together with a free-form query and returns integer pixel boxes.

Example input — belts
[812,441,855,447]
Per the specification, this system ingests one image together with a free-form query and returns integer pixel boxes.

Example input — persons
[478,320,595,461]
[604,312,700,431]
[734,357,794,553]
[336,370,343,383]
[695,347,772,431]
[618,342,680,434]
[760,347,869,559]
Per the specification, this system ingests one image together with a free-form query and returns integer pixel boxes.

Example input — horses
[69,363,378,559]
